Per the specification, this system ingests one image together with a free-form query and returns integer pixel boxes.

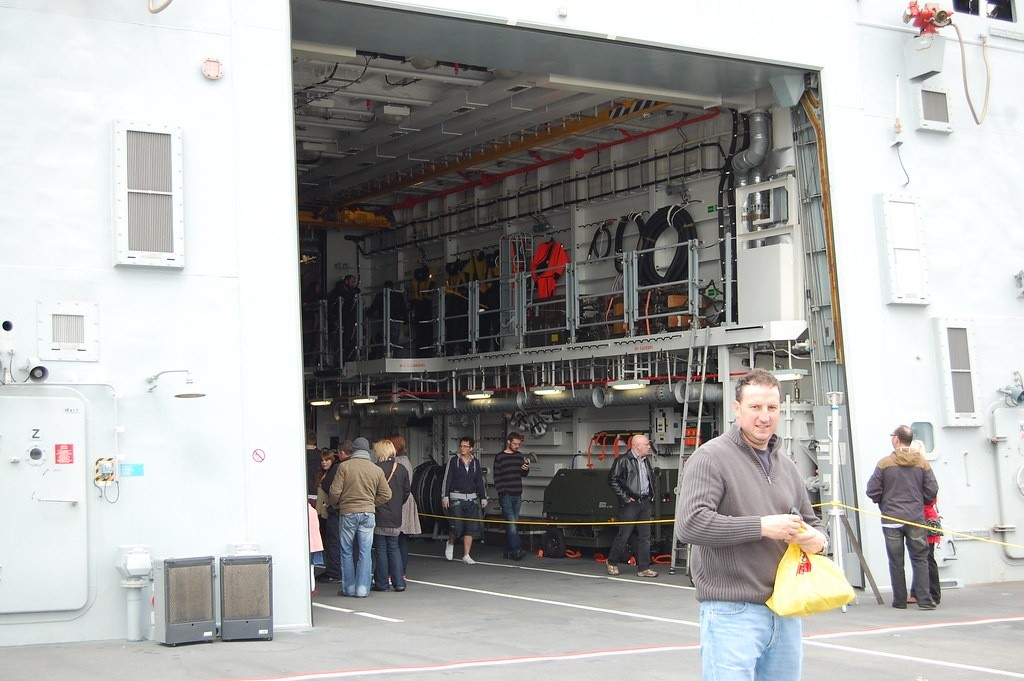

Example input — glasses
[459,445,469,448]
[890,434,898,437]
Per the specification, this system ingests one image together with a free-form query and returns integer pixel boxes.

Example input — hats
[352,437,370,452]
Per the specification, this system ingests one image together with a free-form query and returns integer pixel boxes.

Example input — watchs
[818,537,828,556]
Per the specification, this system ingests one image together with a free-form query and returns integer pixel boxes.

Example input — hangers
[545,234,564,247]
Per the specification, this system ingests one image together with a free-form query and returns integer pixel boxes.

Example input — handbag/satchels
[924,518,944,536]
[765,526,856,616]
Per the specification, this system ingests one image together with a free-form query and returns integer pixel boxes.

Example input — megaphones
[1006,384,1024,404]
[26,358,49,384]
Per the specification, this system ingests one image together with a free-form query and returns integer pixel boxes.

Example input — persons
[324,274,360,364]
[674,370,830,681]
[493,432,531,561]
[364,279,408,357]
[441,437,489,564]
[866,425,939,610]
[906,440,942,604]
[605,435,659,578]
[305,427,422,597]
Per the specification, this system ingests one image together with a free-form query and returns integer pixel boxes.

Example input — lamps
[350,396,378,403]
[530,385,565,395]
[462,390,494,400]
[607,380,650,390]
[145,368,207,399]
[308,398,333,405]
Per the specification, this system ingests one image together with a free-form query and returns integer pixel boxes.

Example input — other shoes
[444,541,454,561]
[462,554,476,565]
[637,569,658,577]
[605,558,619,574]
[918,601,938,608]
[907,597,917,602]
[503,549,526,562]
[338,591,355,597]
[319,575,342,584]
